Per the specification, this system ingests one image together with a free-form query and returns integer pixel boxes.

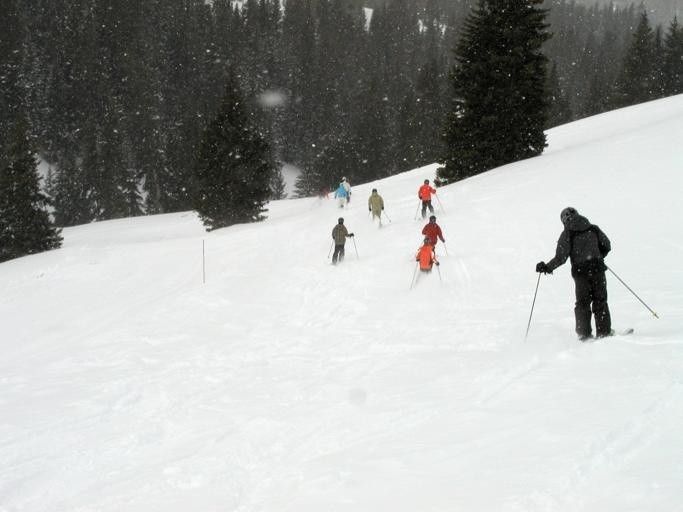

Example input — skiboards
[578,328,634,341]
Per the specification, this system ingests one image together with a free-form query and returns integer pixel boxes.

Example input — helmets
[424,179,429,184]
[372,189,377,192]
[338,217,344,222]
[424,215,437,244]
[560,207,578,224]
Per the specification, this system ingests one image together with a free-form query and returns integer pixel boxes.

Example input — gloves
[369,209,371,211]
[350,233,355,237]
[442,239,445,243]
[420,197,422,199]
[416,259,420,261]
[535,261,553,274]
[436,262,440,266]
[382,207,384,210]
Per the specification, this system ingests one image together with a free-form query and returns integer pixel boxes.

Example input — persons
[329,217,354,266]
[367,187,384,225]
[333,182,347,208]
[417,179,436,220]
[414,237,440,276]
[341,176,351,204]
[535,206,612,340]
[421,216,445,251]
